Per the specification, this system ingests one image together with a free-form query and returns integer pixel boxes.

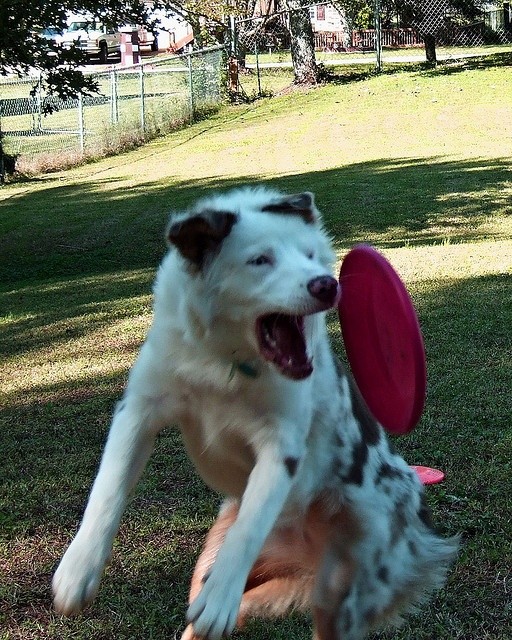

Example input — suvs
[60,18,129,62]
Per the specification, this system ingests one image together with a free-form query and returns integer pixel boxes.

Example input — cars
[118,18,159,52]
[30,25,63,65]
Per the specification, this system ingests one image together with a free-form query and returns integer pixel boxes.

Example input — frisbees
[338,248,427,436]
